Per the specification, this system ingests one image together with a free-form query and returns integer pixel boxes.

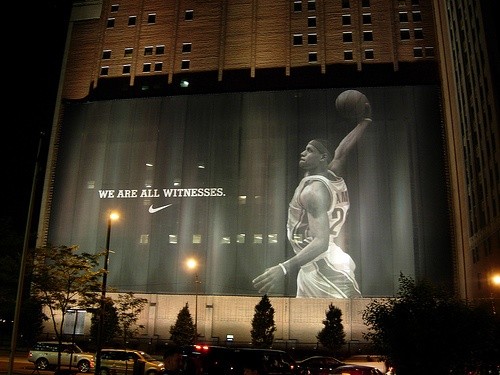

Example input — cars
[293,355,382,375]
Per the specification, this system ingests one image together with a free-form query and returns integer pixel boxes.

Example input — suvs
[95,349,166,375]
[28,341,95,373]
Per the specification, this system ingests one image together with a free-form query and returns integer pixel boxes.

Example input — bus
[178,345,284,375]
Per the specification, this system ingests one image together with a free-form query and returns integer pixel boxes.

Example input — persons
[252,95,373,299]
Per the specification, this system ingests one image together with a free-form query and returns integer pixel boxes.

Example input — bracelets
[364,118,373,123]
[279,264,287,275]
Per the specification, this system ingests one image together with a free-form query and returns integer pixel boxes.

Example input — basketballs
[336,89,367,119]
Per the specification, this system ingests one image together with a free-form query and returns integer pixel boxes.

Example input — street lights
[94,212,119,374]
[187,259,199,341]
